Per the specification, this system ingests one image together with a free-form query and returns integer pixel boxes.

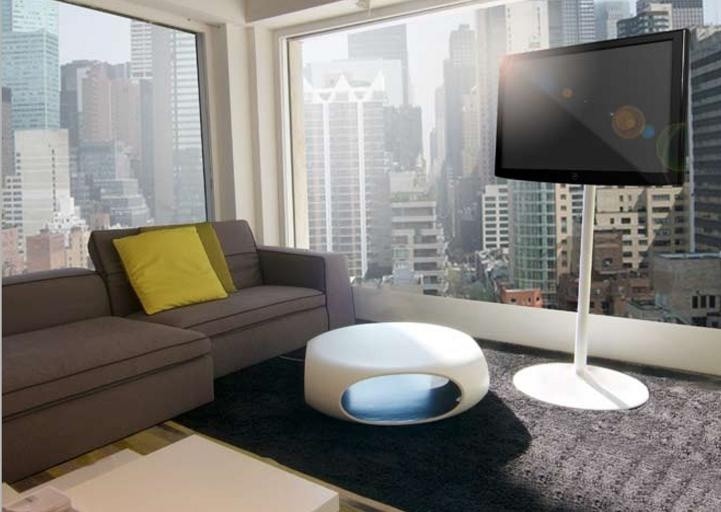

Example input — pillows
[105,219,237,315]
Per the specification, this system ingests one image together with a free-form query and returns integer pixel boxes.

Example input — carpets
[178,347,720,509]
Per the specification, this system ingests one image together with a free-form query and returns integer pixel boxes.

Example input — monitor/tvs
[495,28,690,186]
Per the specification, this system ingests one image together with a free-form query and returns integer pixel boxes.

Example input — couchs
[84,218,358,376]
[2,267,215,488]
